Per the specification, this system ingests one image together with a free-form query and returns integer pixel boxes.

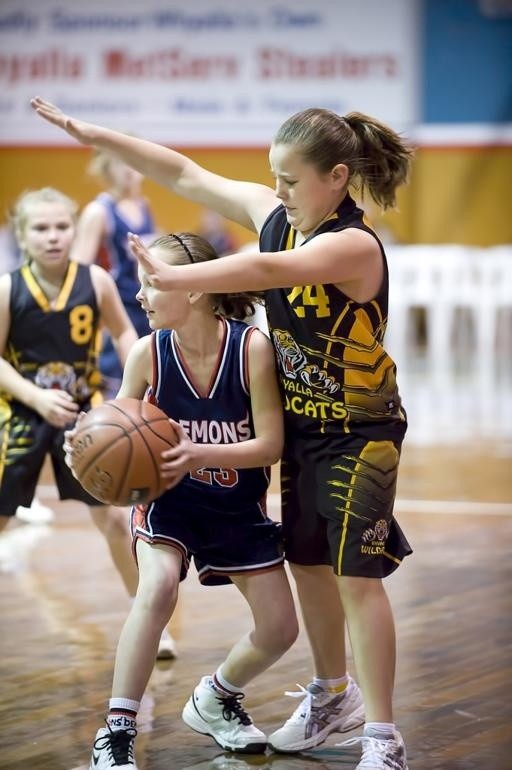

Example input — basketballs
[71,398,178,507]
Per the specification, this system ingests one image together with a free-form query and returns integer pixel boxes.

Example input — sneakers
[17,496,53,523]
[155,627,176,659]
[354,725,409,770]
[268,677,366,753]
[90,726,139,770]
[181,675,267,755]
